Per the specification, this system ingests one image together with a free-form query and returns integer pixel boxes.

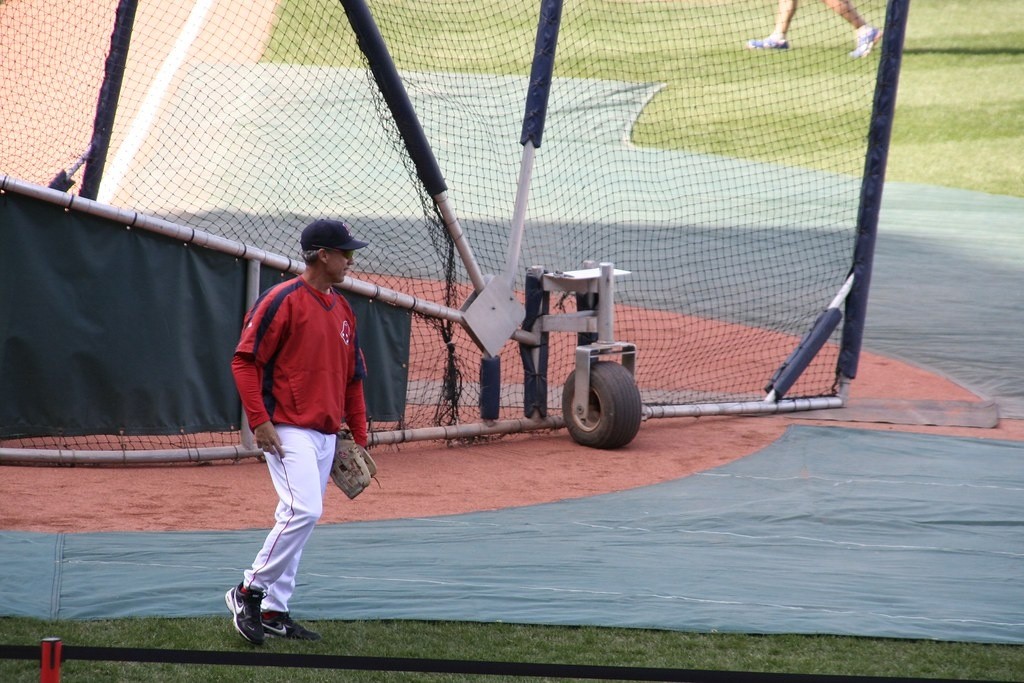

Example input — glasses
[312,243,353,260]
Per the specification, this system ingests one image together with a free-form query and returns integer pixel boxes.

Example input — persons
[746,0,883,58]
[224,219,370,645]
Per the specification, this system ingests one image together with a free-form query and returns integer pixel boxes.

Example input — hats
[300,219,368,253]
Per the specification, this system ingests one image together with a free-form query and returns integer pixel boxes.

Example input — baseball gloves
[324,420,378,501]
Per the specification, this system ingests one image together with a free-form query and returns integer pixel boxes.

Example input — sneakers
[261,610,321,640]
[225,581,265,644]
[749,36,788,50]
[850,27,883,58]
